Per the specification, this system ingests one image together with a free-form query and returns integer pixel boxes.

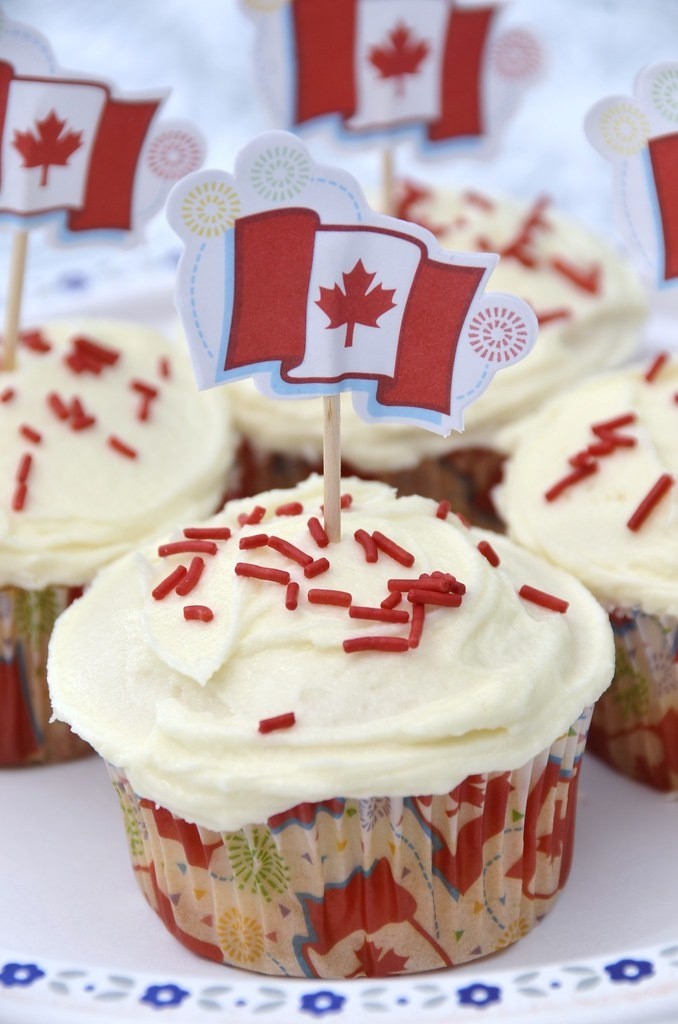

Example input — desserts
[3,171,678,983]
